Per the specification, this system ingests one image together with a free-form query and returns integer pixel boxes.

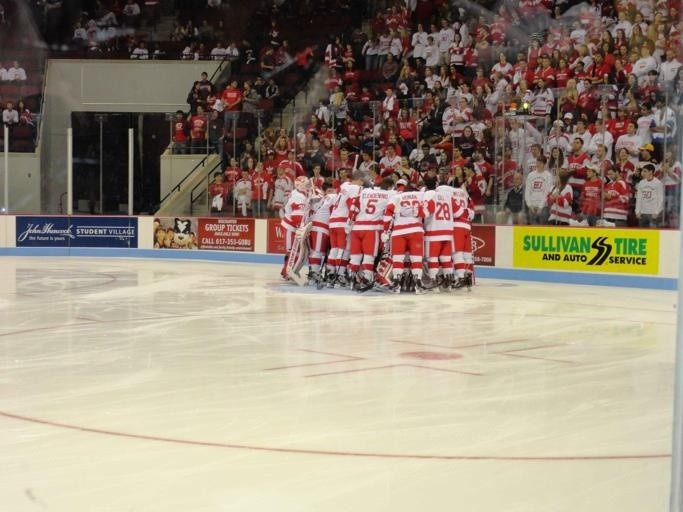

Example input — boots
[308,266,472,293]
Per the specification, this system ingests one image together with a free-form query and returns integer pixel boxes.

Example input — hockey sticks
[315,246,329,291]
[286,188,312,287]
[356,244,383,294]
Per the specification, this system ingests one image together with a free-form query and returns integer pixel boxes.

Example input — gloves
[467,208,475,221]
[380,232,390,243]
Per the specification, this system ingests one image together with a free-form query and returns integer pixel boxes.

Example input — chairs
[0,0,371,160]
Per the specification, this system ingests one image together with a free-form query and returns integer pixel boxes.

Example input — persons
[1,1,683,297]
